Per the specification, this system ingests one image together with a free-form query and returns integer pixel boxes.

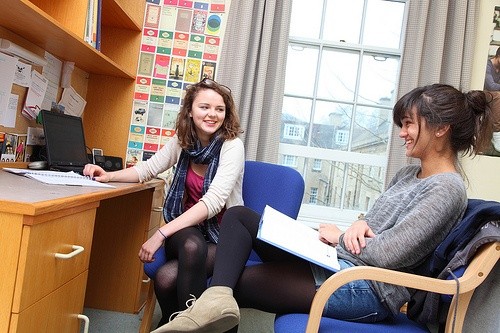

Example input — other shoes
[153,284,243,333]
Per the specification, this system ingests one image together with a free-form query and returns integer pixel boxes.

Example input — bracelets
[157,229,168,240]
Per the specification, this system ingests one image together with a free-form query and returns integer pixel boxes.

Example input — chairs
[140,161,305,333]
[274,198,500,333]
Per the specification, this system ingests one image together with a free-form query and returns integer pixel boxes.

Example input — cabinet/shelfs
[0,0,165,333]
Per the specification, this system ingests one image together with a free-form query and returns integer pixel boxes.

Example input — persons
[485,47,500,93]
[149,83,493,333]
[83,78,246,327]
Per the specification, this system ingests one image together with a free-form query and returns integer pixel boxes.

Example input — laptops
[41,109,86,175]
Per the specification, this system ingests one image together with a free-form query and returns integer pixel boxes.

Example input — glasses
[202,78,232,94]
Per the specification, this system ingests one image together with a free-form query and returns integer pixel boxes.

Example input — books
[2,167,118,188]
[84,0,102,52]
[256,204,340,272]
